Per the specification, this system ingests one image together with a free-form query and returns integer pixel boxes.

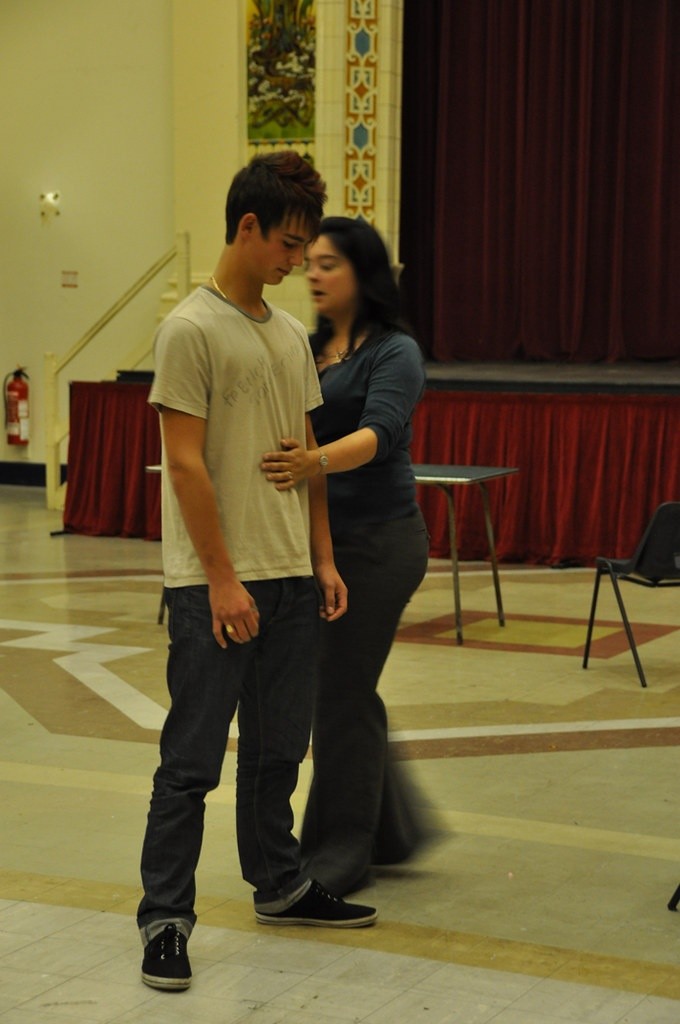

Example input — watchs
[317,448,328,475]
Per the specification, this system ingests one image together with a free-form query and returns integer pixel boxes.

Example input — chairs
[579,500,680,687]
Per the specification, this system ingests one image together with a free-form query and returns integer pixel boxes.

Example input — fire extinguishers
[3,364,29,445]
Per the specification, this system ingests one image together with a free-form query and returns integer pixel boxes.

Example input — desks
[410,461,521,647]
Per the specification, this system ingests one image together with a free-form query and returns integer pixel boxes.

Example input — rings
[225,625,234,634]
[286,472,293,481]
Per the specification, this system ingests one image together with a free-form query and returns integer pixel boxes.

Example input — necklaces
[334,349,347,361]
[210,275,227,301]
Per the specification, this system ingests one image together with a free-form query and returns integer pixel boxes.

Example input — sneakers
[140,922,193,990]
[255,877,379,929]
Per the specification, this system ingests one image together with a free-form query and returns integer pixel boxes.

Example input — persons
[263,214,461,898]
[137,151,380,991]
